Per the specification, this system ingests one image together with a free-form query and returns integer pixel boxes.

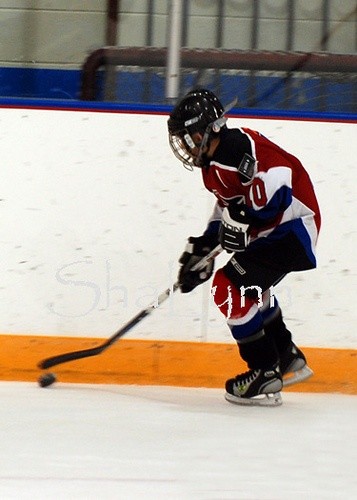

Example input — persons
[167,88,322,409]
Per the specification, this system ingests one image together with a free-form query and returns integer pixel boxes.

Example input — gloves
[216,204,249,252]
[177,236,213,292]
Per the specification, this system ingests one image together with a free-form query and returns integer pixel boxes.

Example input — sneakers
[277,342,314,388]
[224,360,285,406]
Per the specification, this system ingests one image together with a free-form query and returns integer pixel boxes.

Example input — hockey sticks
[33,239,223,367]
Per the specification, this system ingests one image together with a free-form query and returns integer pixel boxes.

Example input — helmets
[167,87,226,165]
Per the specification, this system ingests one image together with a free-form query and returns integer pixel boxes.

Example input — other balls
[37,373,57,389]
